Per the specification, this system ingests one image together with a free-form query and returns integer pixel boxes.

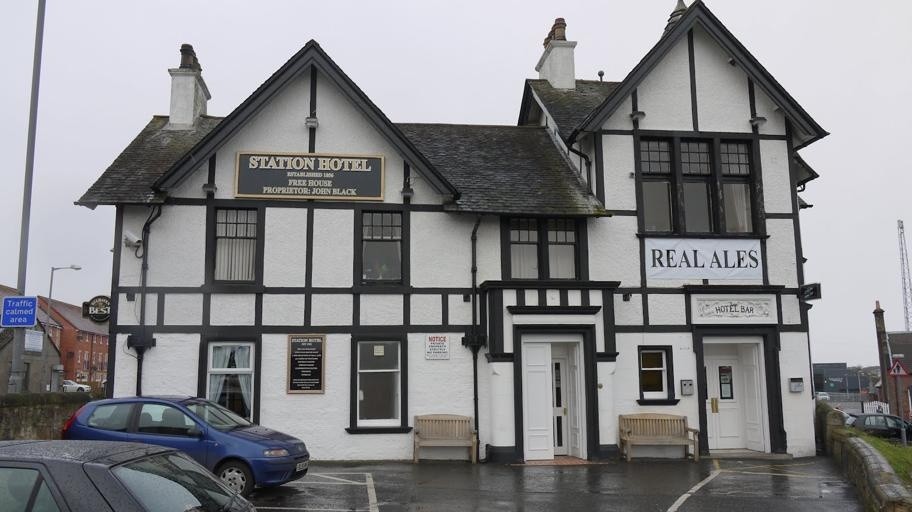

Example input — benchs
[619,413,700,464]
[411,412,479,465]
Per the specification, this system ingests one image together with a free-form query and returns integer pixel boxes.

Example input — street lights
[39,264,82,382]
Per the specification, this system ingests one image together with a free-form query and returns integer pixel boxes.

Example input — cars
[845,413,912,446]
[1,439,257,512]
[61,393,311,498]
[45,380,92,393]
[816,392,830,401]
